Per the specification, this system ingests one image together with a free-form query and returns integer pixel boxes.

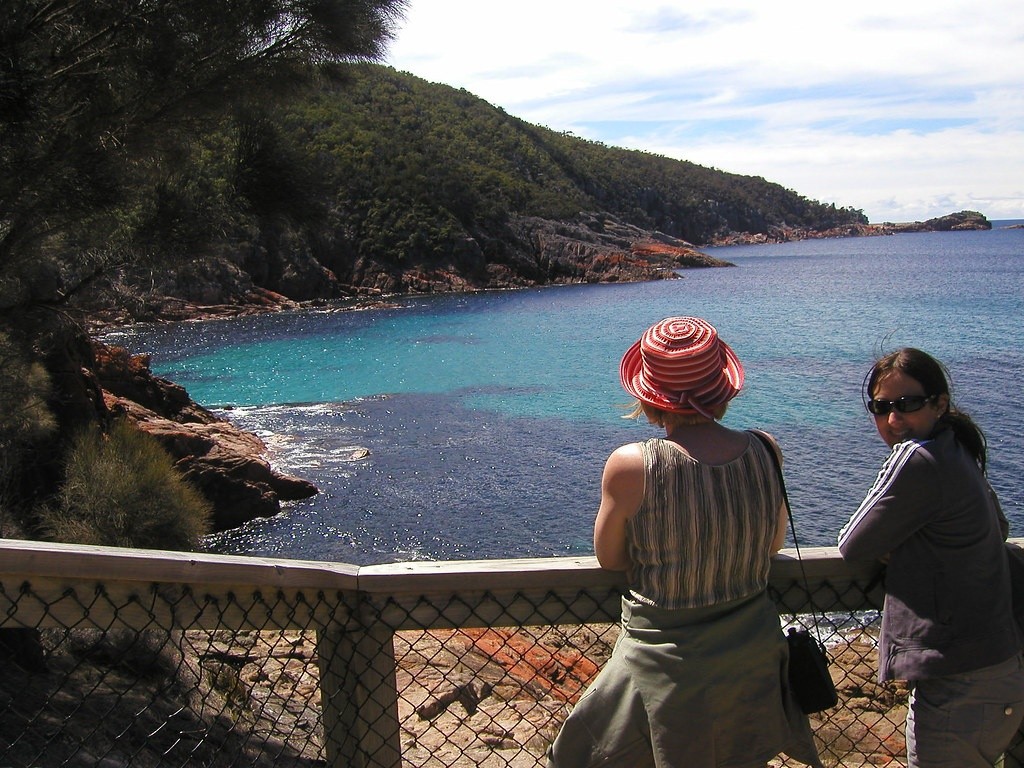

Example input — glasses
[868,393,936,415]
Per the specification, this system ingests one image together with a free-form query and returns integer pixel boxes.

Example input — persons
[838,349,1024,768]
[546,315,825,768]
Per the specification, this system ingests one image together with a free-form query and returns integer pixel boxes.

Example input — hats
[619,316,745,421]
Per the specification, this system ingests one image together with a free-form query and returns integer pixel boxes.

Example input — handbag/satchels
[785,626,837,716]
